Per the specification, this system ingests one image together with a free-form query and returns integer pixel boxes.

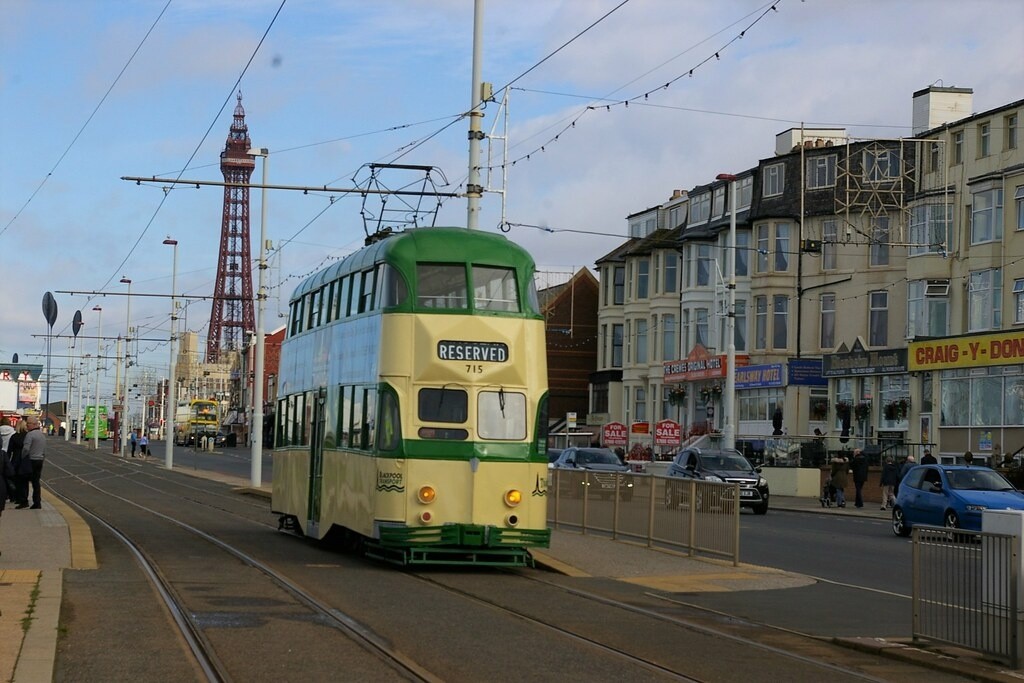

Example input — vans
[72,420,84,440]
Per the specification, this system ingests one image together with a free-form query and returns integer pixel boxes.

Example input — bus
[266,226,551,569]
[174,398,221,452]
[84,405,107,441]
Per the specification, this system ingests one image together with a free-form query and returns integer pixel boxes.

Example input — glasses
[27,422,34,425]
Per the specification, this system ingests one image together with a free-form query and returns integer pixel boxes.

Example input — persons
[810,428,973,510]
[0,417,16,452]
[14,416,48,510]
[130,428,138,458]
[6,420,30,505]
[139,433,149,459]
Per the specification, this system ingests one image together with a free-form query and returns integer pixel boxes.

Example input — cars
[543,448,569,489]
[192,430,226,447]
[890,460,1024,547]
[552,446,635,502]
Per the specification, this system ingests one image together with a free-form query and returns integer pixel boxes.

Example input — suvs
[663,446,771,514]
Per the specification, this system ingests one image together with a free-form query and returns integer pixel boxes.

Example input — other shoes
[15,502,41,509]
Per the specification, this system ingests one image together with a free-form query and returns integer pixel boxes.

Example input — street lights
[162,237,178,472]
[246,146,270,489]
[121,278,132,459]
[91,305,105,449]
